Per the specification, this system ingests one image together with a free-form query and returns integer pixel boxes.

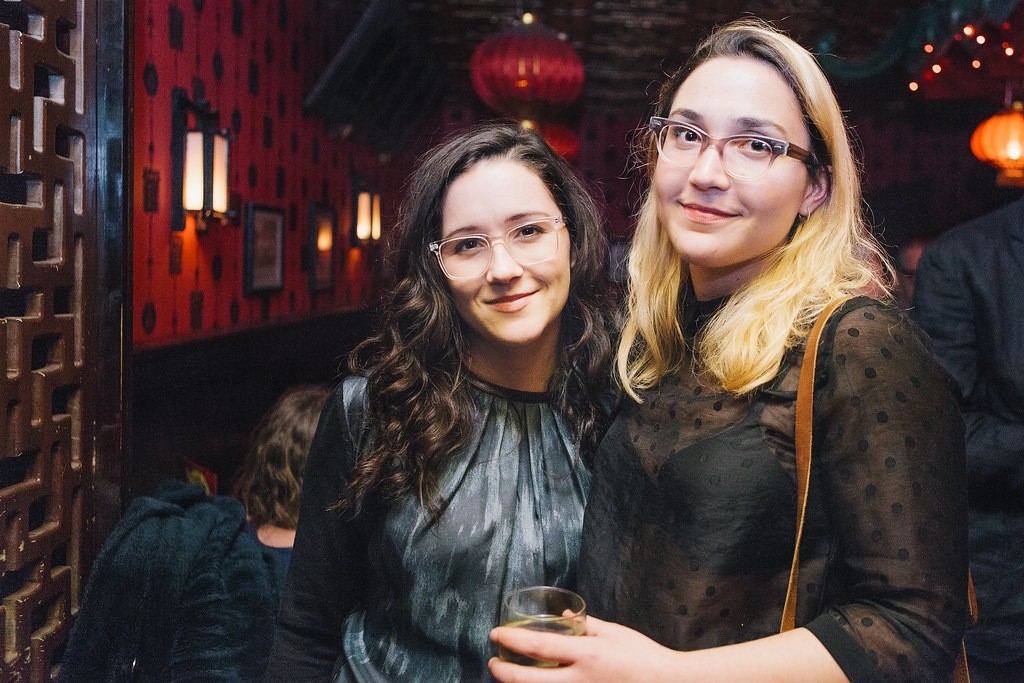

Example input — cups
[499,585,587,675]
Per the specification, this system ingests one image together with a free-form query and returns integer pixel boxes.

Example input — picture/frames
[242,204,289,294]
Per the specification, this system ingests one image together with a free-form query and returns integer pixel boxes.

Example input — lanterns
[471,29,584,116]
[970,111,1024,184]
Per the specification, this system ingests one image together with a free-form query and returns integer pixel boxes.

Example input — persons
[57,389,331,682]
[912,195,1024,683]
[487,23,968,683]
[263,126,644,683]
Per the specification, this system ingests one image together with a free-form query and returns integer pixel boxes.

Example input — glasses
[429,214,569,280]
[649,116,817,180]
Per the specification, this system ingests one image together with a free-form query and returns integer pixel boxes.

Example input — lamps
[166,91,237,232]
